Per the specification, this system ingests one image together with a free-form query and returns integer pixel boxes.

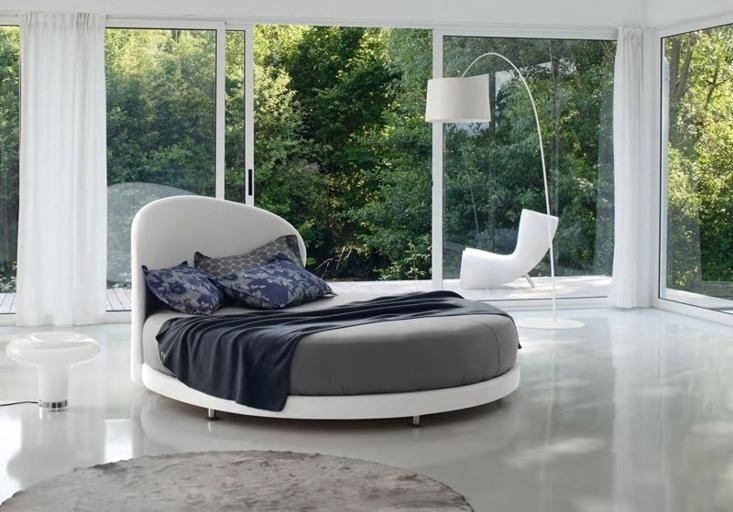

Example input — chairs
[461,209,558,289]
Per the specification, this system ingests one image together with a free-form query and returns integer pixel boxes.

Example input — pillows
[146,235,338,314]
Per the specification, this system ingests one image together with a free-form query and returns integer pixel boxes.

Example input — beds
[129,195,520,429]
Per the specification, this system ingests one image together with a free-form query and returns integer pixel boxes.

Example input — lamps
[424,53,584,331]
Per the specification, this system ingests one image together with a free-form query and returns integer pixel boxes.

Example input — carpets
[0,450,476,510]
